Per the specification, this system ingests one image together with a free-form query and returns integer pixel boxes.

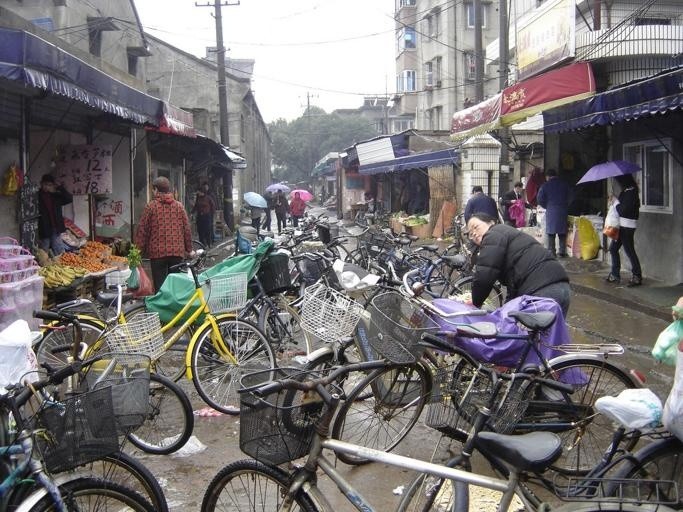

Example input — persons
[289,192,305,226]
[536,168,569,257]
[464,185,499,224]
[466,212,571,321]
[38,173,74,256]
[363,177,427,216]
[136,176,195,295]
[189,186,216,249]
[250,206,261,239]
[604,173,643,287]
[262,191,272,231]
[203,182,216,244]
[500,182,524,227]
[272,189,289,236]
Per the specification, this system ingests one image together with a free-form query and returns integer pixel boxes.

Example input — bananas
[39,263,88,288]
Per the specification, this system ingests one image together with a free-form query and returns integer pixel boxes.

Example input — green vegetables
[128,243,142,271]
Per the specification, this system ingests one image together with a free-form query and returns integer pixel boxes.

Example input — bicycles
[33,251,276,416]
[120,228,313,382]
[201,359,683,512]
[0,361,154,512]
[0,309,169,512]
[0,282,194,454]
[363,255,504,312]
[344,222,463,299]
[333,269,645,478]
[220,213,348,344]
[395,334,683,512]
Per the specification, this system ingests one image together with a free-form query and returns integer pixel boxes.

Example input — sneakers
[627,275,641,286]
[603,272,620,284]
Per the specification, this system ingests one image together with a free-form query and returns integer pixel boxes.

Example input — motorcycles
[280,251,564,436]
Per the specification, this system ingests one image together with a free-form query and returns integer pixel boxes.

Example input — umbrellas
[575,159,643,195]
[266,182,291,205]
[243,191,268,209]
[290,189,313,201]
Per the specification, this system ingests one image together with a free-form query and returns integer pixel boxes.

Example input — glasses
[469,225,478,239]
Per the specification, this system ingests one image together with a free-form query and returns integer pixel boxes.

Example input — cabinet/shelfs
[47,265,118,293]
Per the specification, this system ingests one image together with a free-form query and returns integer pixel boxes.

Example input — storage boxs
[94,279,105,295]
[105,269,131,284]
[69,280,93,299]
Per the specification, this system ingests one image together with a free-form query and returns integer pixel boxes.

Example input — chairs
[215,210,225,240]
